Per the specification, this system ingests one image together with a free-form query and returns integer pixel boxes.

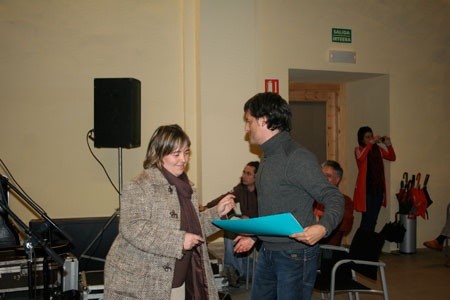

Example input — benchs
[30,216,119,300]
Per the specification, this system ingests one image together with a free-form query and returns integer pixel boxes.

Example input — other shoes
[423,239,444,251]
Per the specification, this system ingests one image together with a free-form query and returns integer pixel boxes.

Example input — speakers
[29,216,120,272]
[94,77,142,148]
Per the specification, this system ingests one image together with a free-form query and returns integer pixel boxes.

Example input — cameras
[380,137,386,143]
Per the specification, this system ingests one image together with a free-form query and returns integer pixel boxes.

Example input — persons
[353,126,396,232]
[422,202,450,252]
[191,159,266,284]
[103,126,237,300]
[309,159,355,267]
[232,92,345,300]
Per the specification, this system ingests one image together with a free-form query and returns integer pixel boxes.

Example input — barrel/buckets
[399,214,416,254]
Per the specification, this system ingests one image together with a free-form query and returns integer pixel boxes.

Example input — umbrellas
[394,172,433,220]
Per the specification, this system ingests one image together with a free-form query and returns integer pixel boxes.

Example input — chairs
[312,226,388,300]
